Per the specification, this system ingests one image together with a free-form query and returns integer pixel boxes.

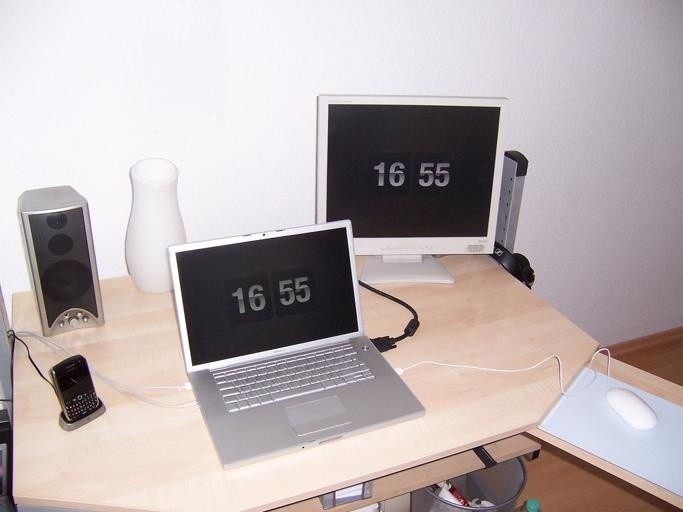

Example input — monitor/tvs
[314,94,510,285]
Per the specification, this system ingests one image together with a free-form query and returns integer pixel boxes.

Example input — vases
[125,165,186,293]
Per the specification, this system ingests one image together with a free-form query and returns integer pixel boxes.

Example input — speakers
[19,185,105,336]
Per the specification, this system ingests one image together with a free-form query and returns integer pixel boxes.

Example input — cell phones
[51,355,99,422]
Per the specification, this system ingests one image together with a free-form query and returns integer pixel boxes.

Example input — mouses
[607,388,659,431]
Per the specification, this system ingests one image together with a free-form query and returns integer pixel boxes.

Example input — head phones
[489,241,534,288]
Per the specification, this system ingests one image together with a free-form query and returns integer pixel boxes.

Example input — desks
[11,237,681,512]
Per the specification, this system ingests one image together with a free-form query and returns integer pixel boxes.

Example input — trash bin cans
[410,455,528,512]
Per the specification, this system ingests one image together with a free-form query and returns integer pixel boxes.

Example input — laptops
[167,219,425,469]
